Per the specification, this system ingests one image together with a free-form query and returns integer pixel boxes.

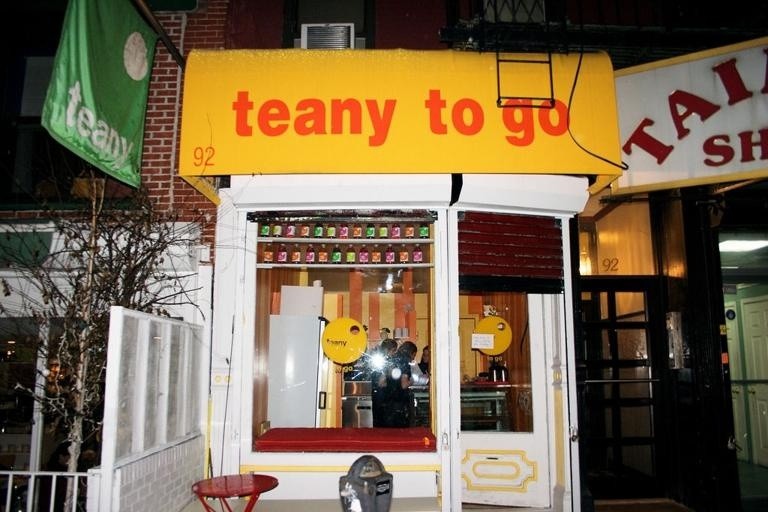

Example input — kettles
[488,365,508,382]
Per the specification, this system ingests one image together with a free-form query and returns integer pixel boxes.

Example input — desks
[192,475,278,512]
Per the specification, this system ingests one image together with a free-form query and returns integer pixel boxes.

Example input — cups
[394,326,408,337]
[312,279,321,287]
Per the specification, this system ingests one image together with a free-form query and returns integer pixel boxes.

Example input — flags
[40,0,159,190]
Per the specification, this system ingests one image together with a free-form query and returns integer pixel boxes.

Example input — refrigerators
[266,314,328,429]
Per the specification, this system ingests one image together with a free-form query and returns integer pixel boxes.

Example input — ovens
[413,392,507,433]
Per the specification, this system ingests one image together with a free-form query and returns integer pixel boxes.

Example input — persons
[353,325,431,429]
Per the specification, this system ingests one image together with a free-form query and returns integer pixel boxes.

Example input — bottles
[286,222,295,238]
[260,222,270,236]
[365,223,430,239]
[352,222,362,238]
[299,223,310,237]
[326,223,337,238]
[338,223,348,239]
[271,221,283,237]
[261,243,423,264]
[314,222,323,239]
